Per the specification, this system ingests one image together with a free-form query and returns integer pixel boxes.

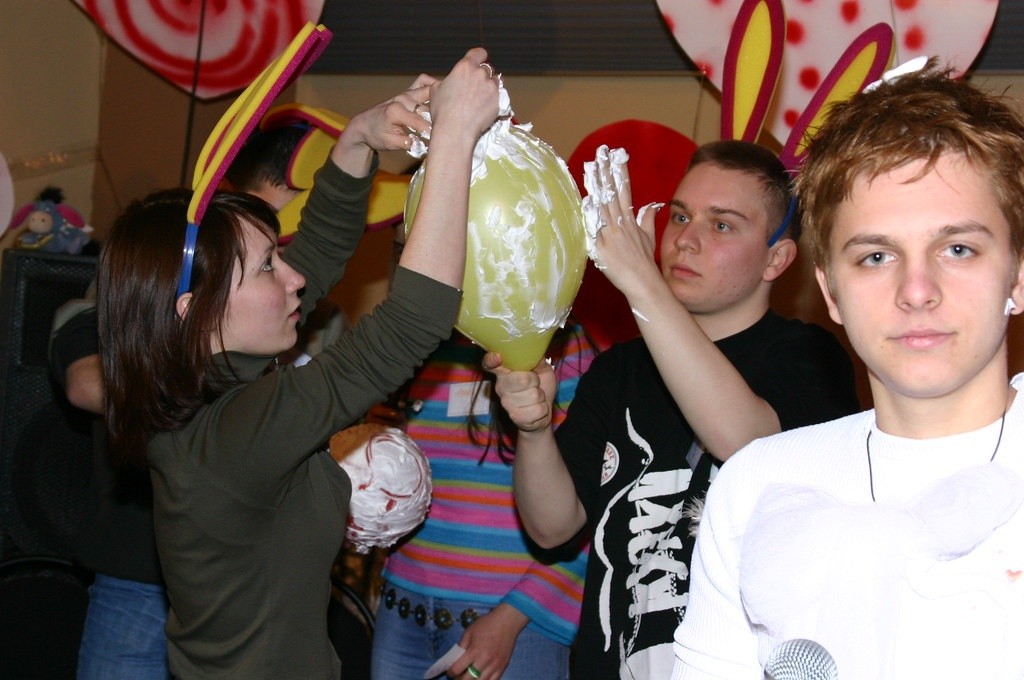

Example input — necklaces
[867,384,1009,502]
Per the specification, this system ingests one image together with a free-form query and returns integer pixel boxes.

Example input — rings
[467,665,480,679]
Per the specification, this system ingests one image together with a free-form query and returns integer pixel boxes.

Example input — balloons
[326,421,432,553]
[402,122,588,369]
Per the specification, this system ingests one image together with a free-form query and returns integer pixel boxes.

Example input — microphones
[765,637,839,680]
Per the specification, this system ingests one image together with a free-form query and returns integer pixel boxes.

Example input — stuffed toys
[16,184,101,258]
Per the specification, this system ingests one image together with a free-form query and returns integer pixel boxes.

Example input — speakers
[1,246,106,607]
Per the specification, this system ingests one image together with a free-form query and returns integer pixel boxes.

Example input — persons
[40,55,1024,680]
